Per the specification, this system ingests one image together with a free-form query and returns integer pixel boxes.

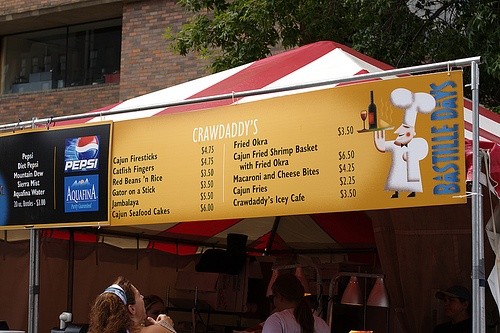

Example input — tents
[10,41,500,253]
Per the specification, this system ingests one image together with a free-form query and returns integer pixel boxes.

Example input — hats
[435,285,472,299]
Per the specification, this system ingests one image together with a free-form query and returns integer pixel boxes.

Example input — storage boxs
[319,263,365,299]
[247,262,273,278]
[171,272,236,311]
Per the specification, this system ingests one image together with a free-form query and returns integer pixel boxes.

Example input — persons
[87,278,174,333]
[262,273,330,333]
[435,285,472,333]
[143,295,165,326]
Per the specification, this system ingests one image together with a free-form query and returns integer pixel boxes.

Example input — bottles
[368,91,377,130]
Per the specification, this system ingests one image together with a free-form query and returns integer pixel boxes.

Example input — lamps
[294,267,311,296]
[266,270,280,296]
[367,277,390,308]
[340,275,364,305]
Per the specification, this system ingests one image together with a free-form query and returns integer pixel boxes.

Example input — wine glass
[361,110,367,130]
